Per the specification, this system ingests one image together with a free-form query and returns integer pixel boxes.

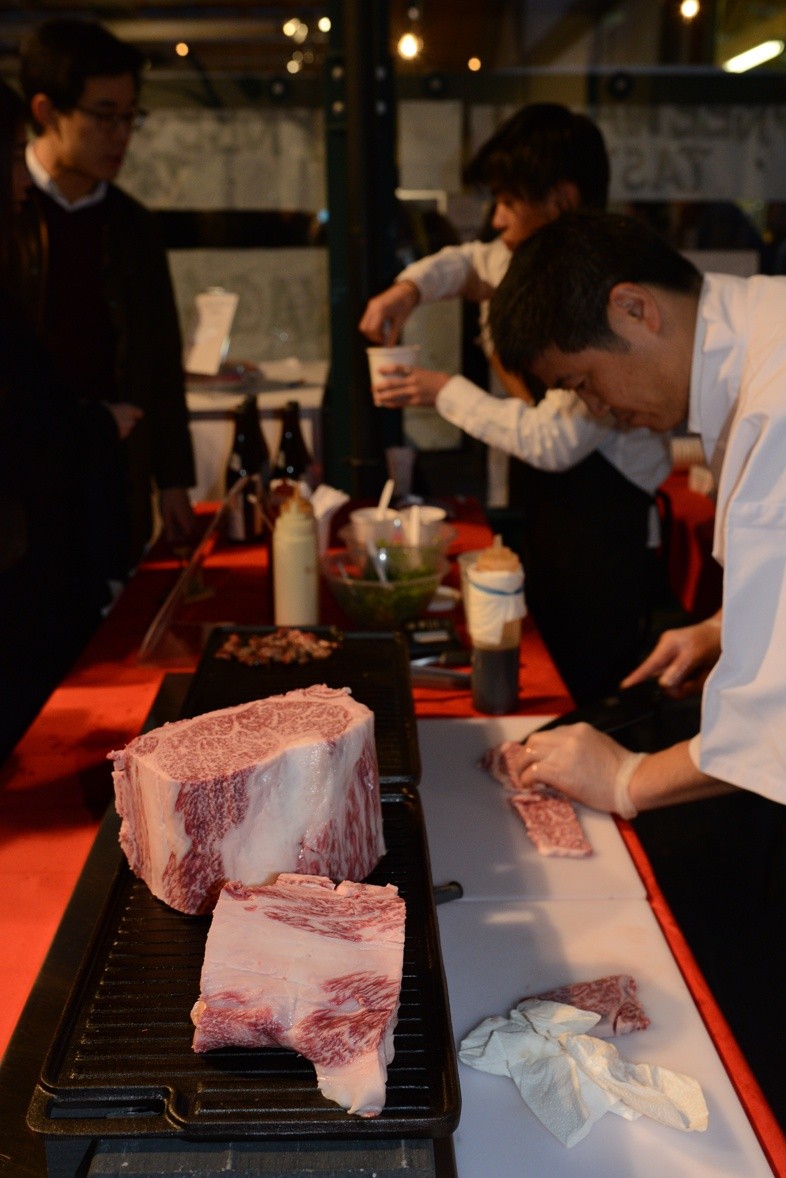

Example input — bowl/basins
[321,544,451,626]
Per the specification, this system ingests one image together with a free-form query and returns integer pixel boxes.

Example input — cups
[457,533,526,716]
[401,503,447,547]
[349,508,397,543]
[366,345,420,409]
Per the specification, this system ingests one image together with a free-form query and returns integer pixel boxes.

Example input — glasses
[42,90,150,132]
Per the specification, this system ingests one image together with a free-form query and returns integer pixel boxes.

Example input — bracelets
[616,750,649,818]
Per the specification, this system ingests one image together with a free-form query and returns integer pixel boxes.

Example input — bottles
[226,395,313,545]
[273,483,320,626]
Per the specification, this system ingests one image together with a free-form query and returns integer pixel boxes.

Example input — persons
[360,101,673,682]
[0,80,139,751]
[487,213,785,1095]
[1,14,196,637]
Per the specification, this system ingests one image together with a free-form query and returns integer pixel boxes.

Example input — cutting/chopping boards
[414,704,668,901]
[430,894,778,1178]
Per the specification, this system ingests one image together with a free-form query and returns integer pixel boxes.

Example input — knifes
[520,666,698,747]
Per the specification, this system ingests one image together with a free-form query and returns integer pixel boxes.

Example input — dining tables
[2,489,785,1177]
